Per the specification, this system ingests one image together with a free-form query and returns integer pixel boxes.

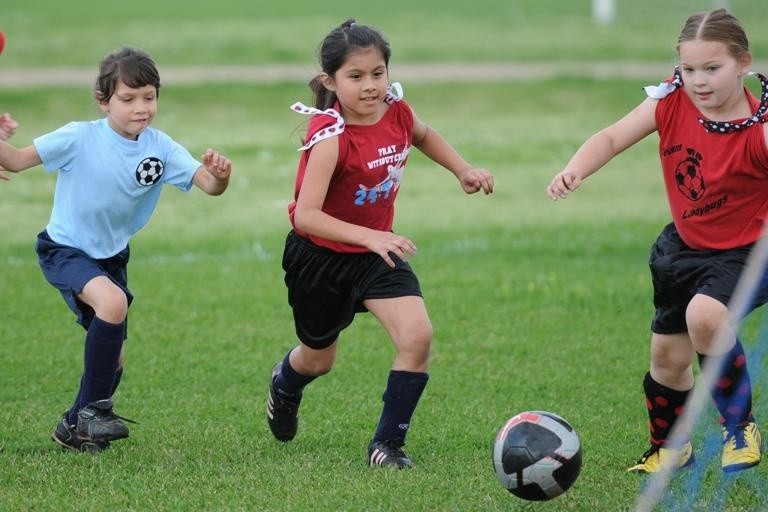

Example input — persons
[547,7,768,474]
[267,19,493,471]
[0,48,231,451]
[0,113,19,181]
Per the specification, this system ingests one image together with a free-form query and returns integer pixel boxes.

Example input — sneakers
[721,421,762,473]
[267,362,302,442]
[628,441,697,474]
[367,439,414,471]
[51,399,129,455]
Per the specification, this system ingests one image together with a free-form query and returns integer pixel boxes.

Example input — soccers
[492,411,582,501]
